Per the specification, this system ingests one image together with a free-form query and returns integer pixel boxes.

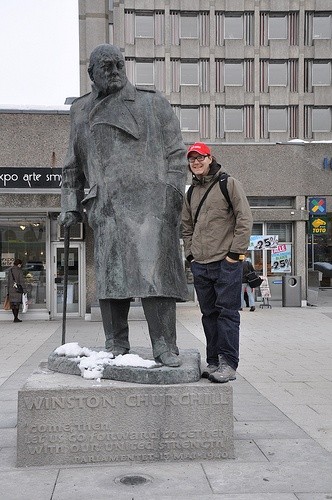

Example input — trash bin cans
[282,275,301,307]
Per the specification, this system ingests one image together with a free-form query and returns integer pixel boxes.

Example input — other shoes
[14,319,22,322]
[249,307,255,311]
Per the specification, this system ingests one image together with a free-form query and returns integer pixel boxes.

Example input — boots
[209,355,236,383]
[202,364,217,378]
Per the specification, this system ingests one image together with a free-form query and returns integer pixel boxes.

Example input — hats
[186,142,210,156]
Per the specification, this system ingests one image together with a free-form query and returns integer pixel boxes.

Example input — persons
[57,42,194,367]
[6,258,28,323]
[239,260,256,312]
[180,142,255,384]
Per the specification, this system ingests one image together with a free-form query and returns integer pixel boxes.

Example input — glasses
[189,156,207,162]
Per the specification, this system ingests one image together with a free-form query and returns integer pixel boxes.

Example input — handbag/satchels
[246,262,262,288]
[4,297,11,310]
[14,283,23,293]
[22,292,28,313]
